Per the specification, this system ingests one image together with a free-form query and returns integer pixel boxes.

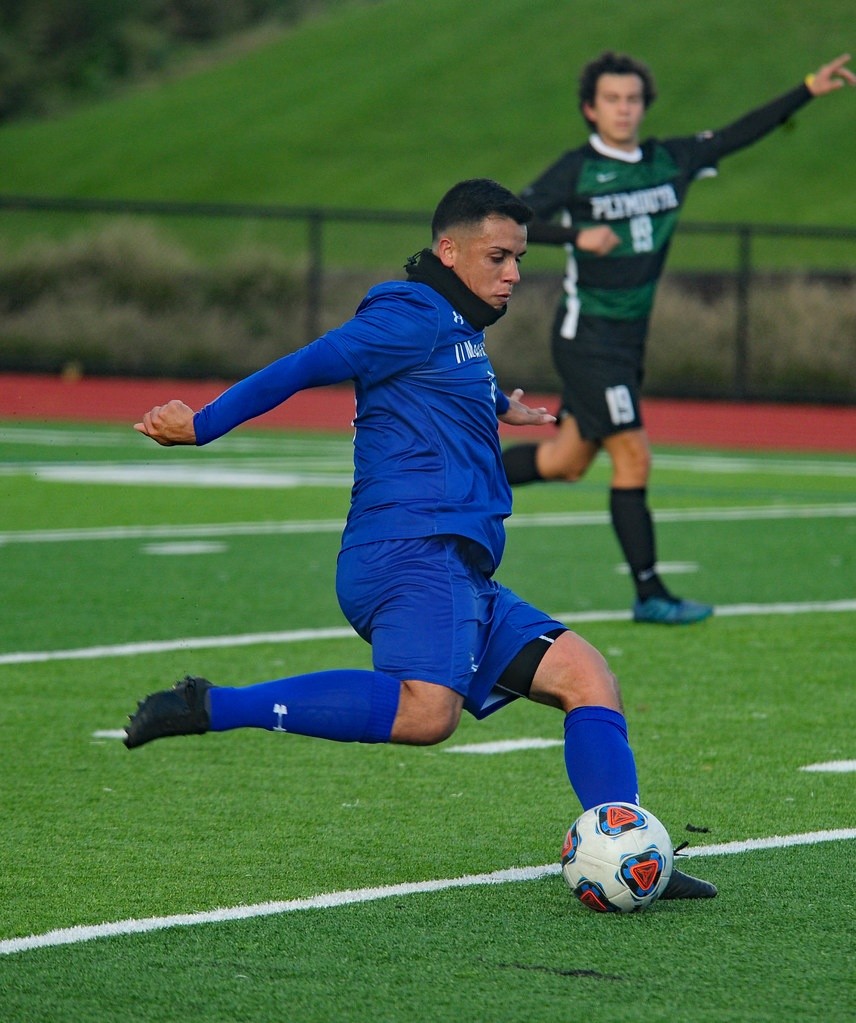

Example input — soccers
[561,802,673,913]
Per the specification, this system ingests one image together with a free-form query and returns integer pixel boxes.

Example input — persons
[513,49,856,624]
[122,179,720,900]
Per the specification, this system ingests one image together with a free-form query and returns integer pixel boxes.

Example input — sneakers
[658,841,718,899]
[633,596,714,625]
[122,675,219,751]
